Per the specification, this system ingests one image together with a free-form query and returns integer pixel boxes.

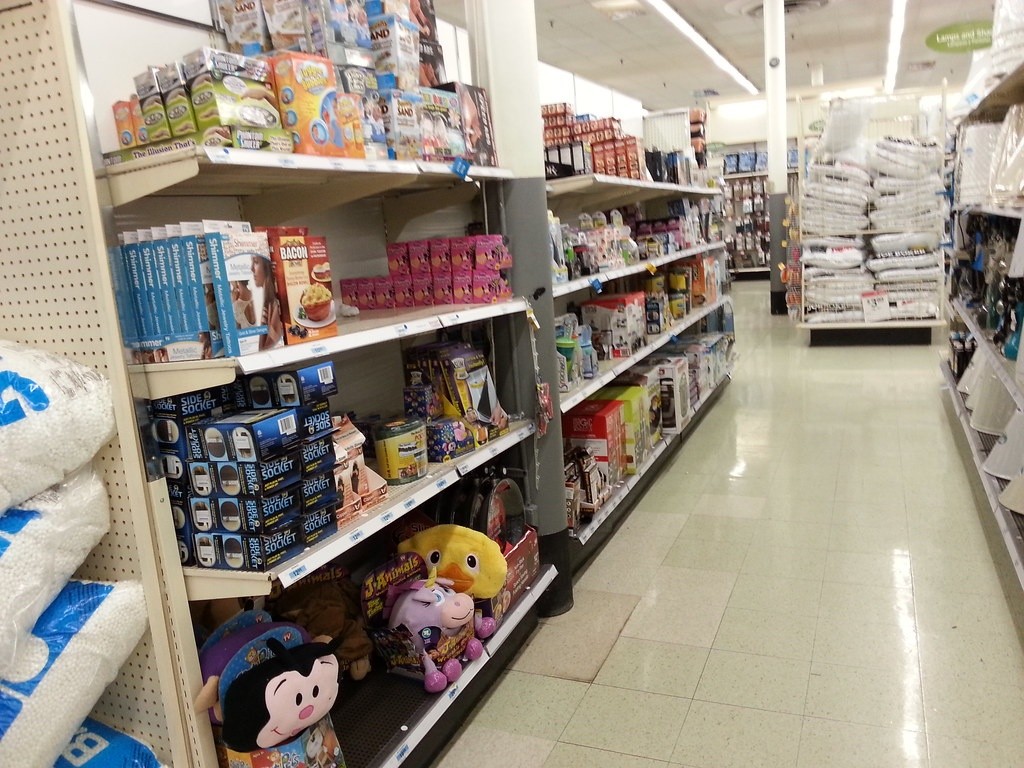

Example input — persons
[231,255,281,352]
[204,284,220,330]
[198,332,212,359]
[131,348,168,364]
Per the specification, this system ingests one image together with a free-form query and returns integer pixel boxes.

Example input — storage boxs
[564,253,728,537]
[947,329,978,382]
[107,0,540,684]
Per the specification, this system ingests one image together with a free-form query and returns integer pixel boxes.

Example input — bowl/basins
[300,294,330,321]
[314,272,325,278]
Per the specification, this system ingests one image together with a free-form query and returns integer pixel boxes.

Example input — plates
[293,300,336,328]
[311,271,331,283]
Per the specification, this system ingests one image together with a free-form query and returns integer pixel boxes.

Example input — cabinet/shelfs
[939,66,1024,591]
[106,143,560,768]
[795,224,947,346]
[542,102,808,576]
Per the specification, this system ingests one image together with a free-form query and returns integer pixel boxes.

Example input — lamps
[811,61,824,88]
[953,104,1024,517]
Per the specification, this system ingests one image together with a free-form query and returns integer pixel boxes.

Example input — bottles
[948,331,977,377]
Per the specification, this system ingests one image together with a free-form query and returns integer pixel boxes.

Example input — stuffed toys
[195,522,507,754]
